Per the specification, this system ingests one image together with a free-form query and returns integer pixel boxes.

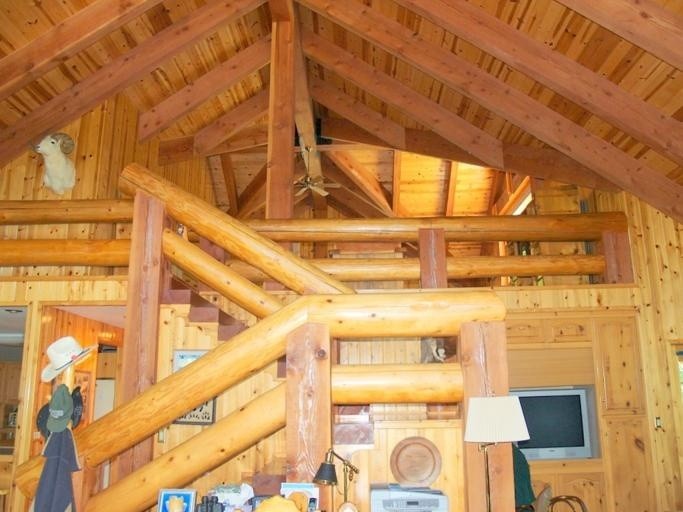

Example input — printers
[370,484,449,512]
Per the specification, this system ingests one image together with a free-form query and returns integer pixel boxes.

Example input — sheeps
[33,131,77,192]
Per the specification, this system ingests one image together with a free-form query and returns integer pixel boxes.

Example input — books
[279,482,316,489]
[280,487,319,512]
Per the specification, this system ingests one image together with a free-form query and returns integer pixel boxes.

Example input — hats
[36,385,84,444]
[39,335,99,383]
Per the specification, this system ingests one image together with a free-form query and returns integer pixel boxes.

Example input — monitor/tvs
[507,384,601,460]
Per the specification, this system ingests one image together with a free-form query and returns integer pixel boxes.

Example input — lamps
[464,395,530,512]
[312,448,359,503]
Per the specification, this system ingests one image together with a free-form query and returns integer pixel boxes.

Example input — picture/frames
[170,348,216,425]
[157,488,196,512]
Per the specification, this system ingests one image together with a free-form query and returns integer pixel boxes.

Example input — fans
[293,145,341,197]
